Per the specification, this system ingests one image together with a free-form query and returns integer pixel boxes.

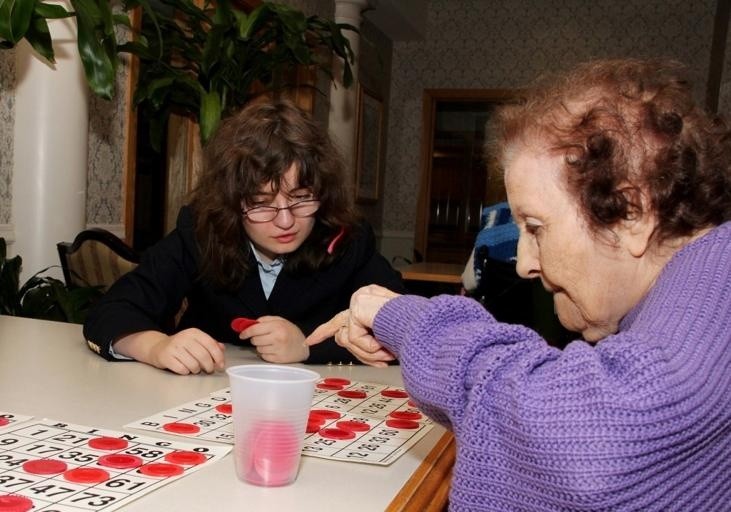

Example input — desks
[391,256,470,282]
[0,310,469,509]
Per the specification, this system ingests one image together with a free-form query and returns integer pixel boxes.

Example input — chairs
[56,230,163,305]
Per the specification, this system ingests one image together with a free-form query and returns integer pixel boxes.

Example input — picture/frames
[356,79,387,207]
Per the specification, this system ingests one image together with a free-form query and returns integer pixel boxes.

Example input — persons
[83,97,407,376]
[303,57,731,512]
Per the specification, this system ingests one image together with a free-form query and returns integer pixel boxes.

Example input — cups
[226,363,321,488]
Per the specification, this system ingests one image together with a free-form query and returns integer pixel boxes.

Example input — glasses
[241,199,320,223]
